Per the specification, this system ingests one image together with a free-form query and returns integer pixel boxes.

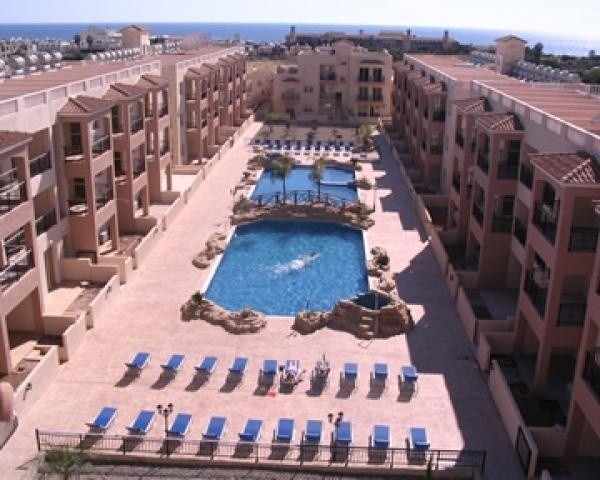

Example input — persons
[281,360,308,386]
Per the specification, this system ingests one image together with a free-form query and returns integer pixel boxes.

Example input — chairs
[301,418,324,443]
[410,427,431,452]
[199,415,228,439]
[166,413,193,439]
[253,139,368,159]
[126,409,156,436]
[124,350,419,384]
[330,422,353,445]
[237,418,265,443]
[274,417,298,442]
[85,407,117,433]
[370,424,391,450]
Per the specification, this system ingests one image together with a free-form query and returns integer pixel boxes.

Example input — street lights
[155,403,174,459]
[371,183,377,214]
[325,410,345,462]
